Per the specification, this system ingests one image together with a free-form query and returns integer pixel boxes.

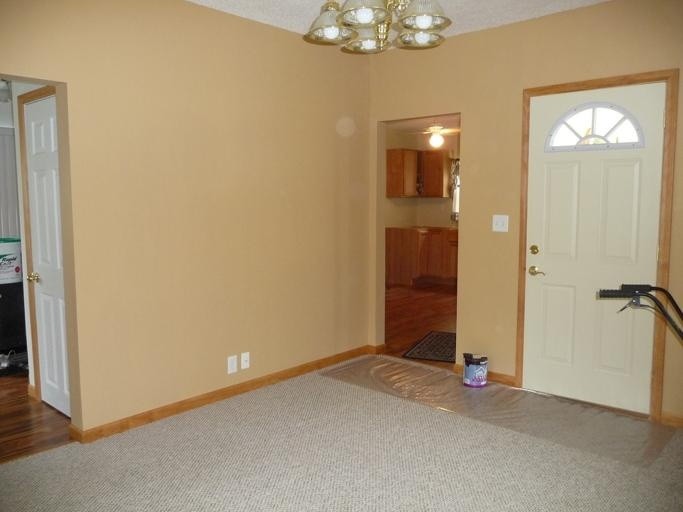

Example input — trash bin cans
[463,353,489,387]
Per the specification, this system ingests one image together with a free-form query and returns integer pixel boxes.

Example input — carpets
[402,330,456,363]
[0,352,682,512]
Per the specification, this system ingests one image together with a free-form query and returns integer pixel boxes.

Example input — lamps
[302,0,453,54]
[422,125,459,148]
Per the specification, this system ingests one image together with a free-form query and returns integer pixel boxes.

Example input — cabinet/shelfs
[428,227,459,287]
[419,150,454,198]
[385,149,420,198]
[384,227,429,288]
